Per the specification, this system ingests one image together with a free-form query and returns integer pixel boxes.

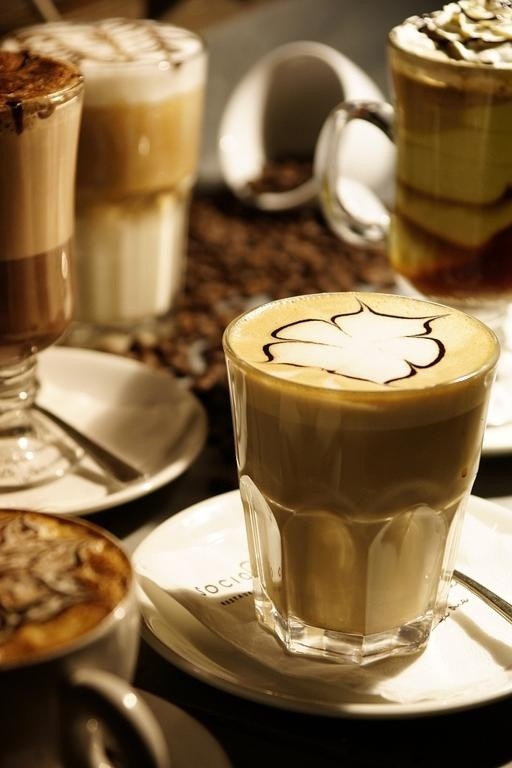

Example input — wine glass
[0,46,79,360]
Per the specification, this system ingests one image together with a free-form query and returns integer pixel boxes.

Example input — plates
[383,280,512,454]
[129,682,230,766]
[0,344,205,515]
[132,484,512,722]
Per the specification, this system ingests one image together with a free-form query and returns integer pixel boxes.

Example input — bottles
[0,343,43,489]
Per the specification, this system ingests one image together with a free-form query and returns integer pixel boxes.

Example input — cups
[1,507,174,768]
[26,14,209,333]
[220,289,499,664]
[306,5,511,332]
[217,39,391,216]
